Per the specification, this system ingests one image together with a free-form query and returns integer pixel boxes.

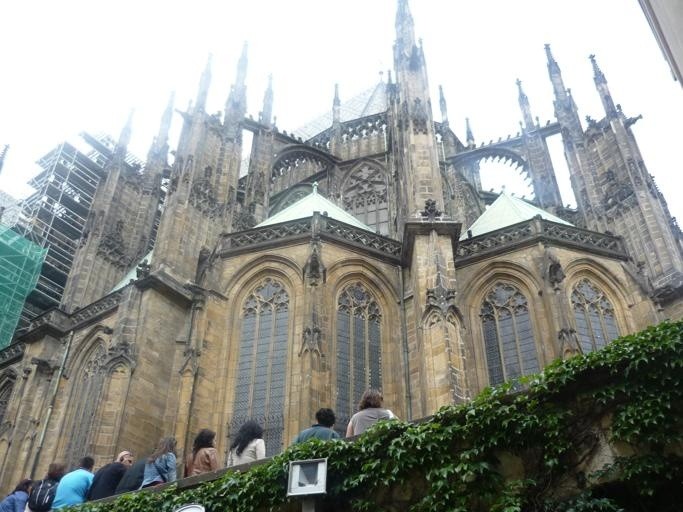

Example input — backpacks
[29,479,59,512]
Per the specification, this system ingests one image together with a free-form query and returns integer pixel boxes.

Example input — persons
[115,448,157,493]
[87,451,136,501]
[50,456,88,509]
[184,428,218,477]
[24,480,42,512]
[295,408,340,445]
[28,463,65,512]
[142,436,177,488]
[227,420,265,468]
[0,479,34,512]
[346,388,400,438]
